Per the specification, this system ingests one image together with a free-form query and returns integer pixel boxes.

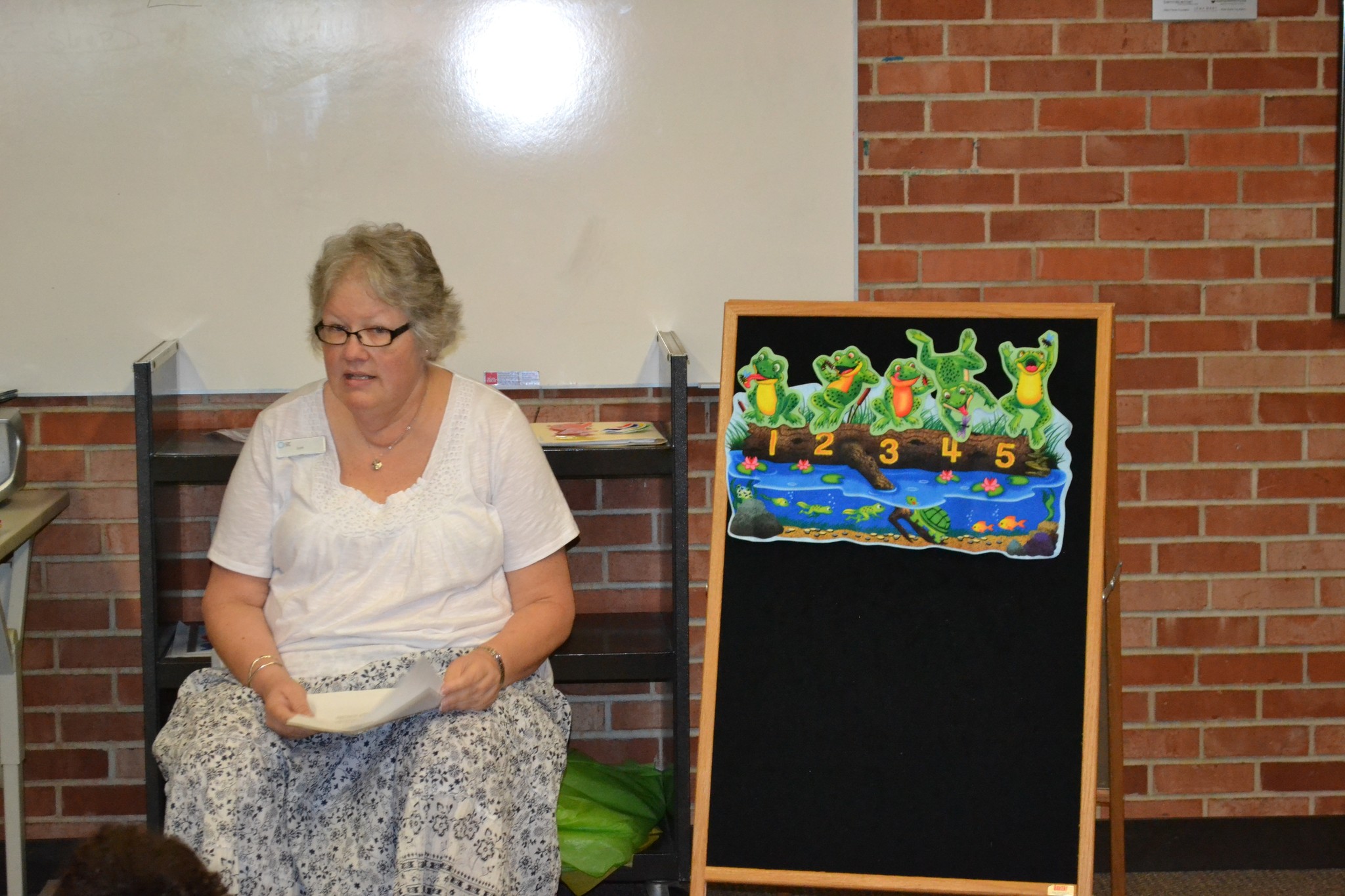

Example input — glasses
[314,318,414,347]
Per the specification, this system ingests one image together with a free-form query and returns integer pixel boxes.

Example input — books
[527,421,668,447]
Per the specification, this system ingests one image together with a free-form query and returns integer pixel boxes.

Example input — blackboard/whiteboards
[689,298,1115,895]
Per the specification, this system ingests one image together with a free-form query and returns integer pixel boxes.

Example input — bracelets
[247,655,288,688]
[473,645,504,691]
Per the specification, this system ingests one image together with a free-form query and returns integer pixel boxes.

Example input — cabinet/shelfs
[133,331,696,896]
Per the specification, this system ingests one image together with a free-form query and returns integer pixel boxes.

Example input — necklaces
[354,365,429,472]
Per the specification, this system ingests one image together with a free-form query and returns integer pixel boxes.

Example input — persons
[147,220,583,896]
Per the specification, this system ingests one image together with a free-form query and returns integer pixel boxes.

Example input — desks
[0,487,71,896]
